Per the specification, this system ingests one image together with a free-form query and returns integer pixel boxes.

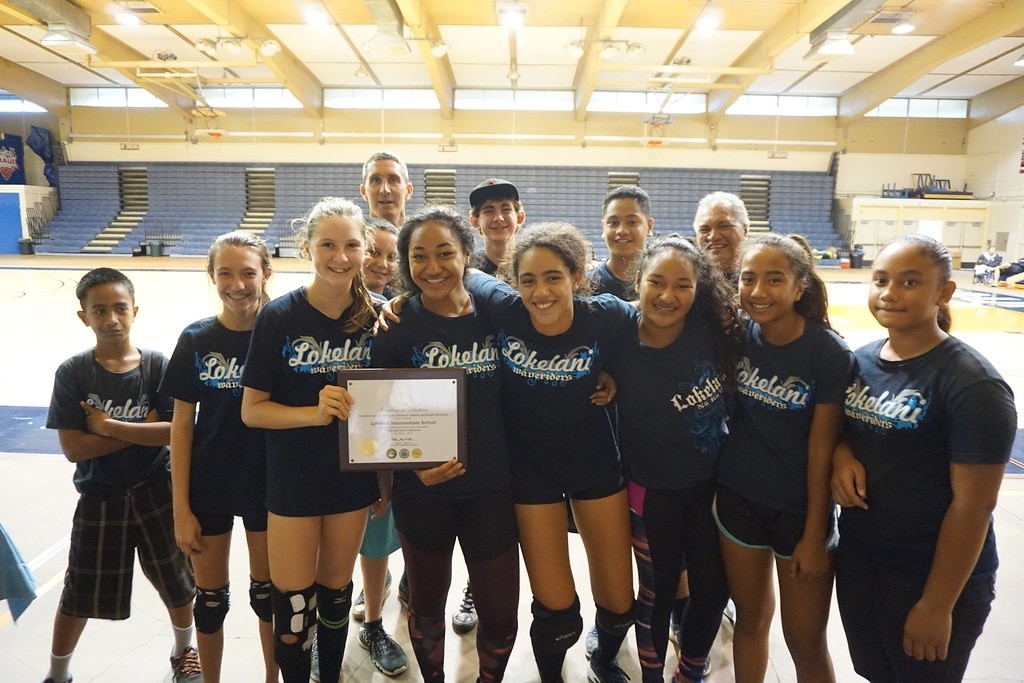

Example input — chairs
[973,257,1002,284]
[881,173,951,198]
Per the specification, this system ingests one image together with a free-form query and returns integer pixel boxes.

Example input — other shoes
[587,647,629,683]
[585,626,600,658]
[669,617,712,676]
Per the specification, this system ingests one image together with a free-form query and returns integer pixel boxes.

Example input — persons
[352,149,414,619]
[575,184,654,311]
[712,232,858,683]
[369,208,616,683]
[157,231,280,683]
[988,257,1024,289]
[452,179,525,629]
[977,246,1003,284]
[693,190,750,628]
[42,268,204,683]
[604,233,728,682]
[242,196,392,683]
[368,222,738,683]
[973,259,986,284]
[362,215,411,677]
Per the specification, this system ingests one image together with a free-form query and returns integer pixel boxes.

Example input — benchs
[32,162,848,266]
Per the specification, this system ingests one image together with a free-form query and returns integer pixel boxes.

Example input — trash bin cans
[19,241,33,254]
[149,242,163,256]
[850,252,863,268]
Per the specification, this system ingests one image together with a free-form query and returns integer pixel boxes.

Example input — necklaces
[832,233,1017,683]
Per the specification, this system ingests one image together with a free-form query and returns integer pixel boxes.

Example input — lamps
[568,39,644,59]
[194,38,281,57]
[891,19,915,35]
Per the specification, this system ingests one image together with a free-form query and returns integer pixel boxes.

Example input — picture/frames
[336,367,469,473]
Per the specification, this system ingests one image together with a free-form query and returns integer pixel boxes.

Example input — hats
[469,178,519,208]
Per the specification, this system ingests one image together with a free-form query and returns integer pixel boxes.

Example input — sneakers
[398,573,409,609]
[310,627,343,681]
[353,568,392,619]
[41,671,74,683]
[170,644,204,683]
[357,611,409,675]
[452,578,478,633]
